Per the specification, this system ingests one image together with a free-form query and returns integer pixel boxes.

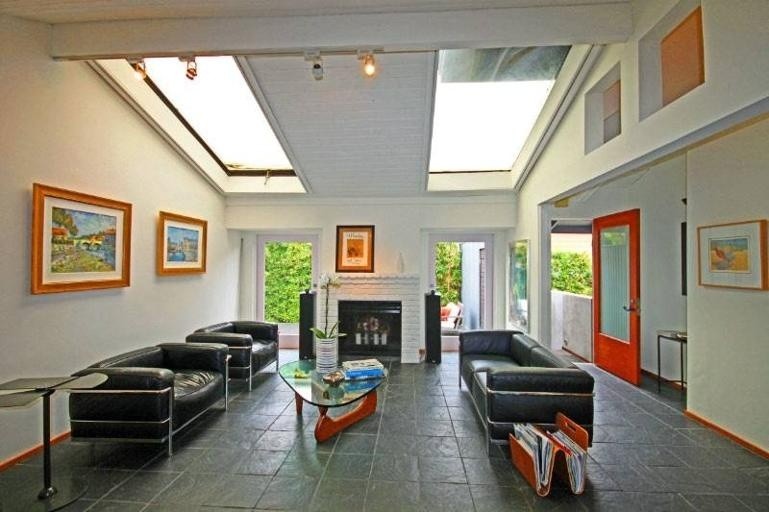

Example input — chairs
[440,302,464,330]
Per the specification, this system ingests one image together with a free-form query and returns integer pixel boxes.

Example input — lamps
[177,56,199,81]
[132,58,149,81]
[312,65,325,82]
[361,53,378,78]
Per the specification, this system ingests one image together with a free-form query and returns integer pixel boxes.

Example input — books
[340,379,384,399]
[509,419,587,495]
[340,358,387,381]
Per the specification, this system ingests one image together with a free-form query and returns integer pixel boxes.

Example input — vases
[315,340,338,372]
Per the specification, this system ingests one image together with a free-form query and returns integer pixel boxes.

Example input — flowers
[308,272,353,339]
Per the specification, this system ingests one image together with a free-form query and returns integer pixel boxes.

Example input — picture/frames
[335,224,376,274]
[29,182,134,296]
[156,210,208,277]
[696,218,769,292]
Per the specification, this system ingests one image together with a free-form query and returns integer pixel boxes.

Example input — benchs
[68,342,233,456]
[185,320,279,392]
[458,330,596,458]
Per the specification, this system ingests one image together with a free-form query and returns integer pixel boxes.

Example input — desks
[0,372,109,512]
[654,328,687,393]
